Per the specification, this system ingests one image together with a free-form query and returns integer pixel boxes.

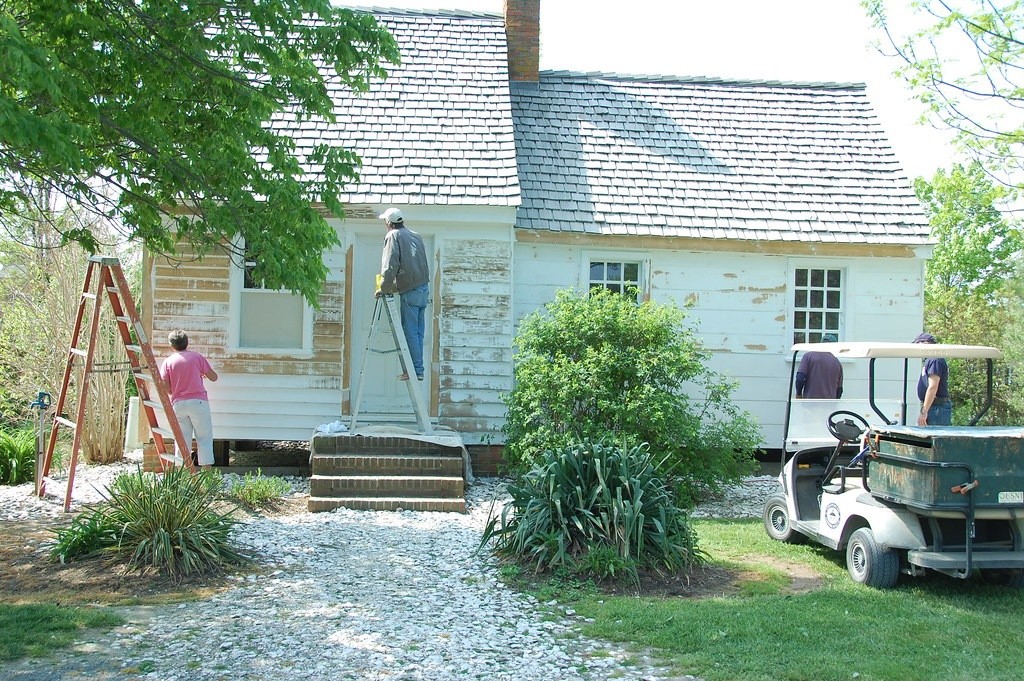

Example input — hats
[911,333,936,342]
[821,334,837,342]
[379,208,403,223]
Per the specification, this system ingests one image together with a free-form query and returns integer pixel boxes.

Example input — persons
[160,330,218,470]
[374,207,430,382]
[796,333,843,398]
[911,333,952,426]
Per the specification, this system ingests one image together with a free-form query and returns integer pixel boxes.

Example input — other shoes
[397,373,424,381]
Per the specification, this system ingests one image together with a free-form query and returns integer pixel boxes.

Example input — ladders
[38,254,204,510]
[347,288,434,437]
